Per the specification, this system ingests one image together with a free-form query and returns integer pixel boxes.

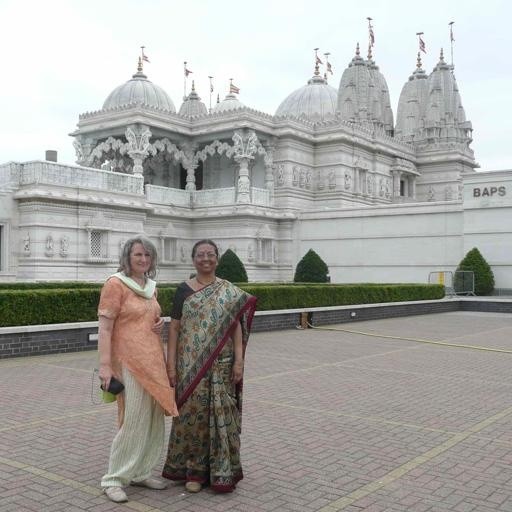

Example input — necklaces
[197,277,208,287]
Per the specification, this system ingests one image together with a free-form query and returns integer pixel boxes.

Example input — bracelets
[167,366,178,372]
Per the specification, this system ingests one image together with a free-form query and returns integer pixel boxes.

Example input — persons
[161,238,258,495]
[96,235,180,503]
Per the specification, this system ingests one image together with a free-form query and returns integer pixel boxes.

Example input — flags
[142,14,455,104]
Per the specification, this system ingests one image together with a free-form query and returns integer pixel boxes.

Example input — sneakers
[105,487,128,502]
[131,479,166,489]
[185,482,201,492]
